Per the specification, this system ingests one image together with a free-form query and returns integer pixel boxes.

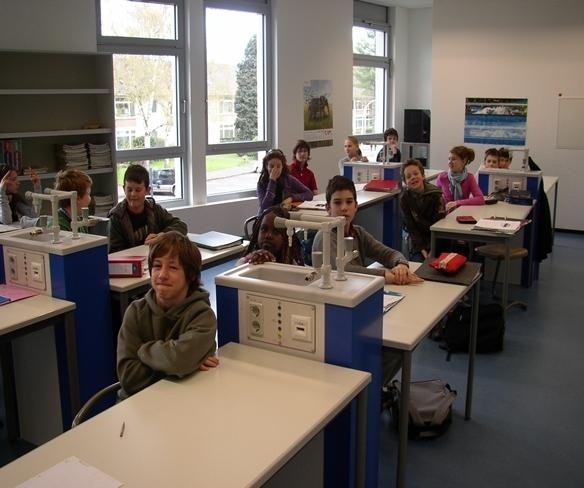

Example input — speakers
[404,109,431,143]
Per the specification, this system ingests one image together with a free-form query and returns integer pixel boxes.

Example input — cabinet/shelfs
[0,49,117,216]
[399,142,430,167]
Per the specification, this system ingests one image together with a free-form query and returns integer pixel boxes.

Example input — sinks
[215,261,385,307]
[0,225,107,256]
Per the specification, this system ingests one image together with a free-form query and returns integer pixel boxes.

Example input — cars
[150,168,177,194]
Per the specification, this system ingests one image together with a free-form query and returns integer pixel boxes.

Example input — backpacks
[391,378,457,441]
[439,303,504,360]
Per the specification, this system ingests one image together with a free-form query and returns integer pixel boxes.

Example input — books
[297,200,328,210]
[1,136,49,177]
[187,231,243,250]
[469,215,532,238]
[55,141,114,219]
[383,291,406,313]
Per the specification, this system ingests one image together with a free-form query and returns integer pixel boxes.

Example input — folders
[191,229,245,252]
[109,254,150,278]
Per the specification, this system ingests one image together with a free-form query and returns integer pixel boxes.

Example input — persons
[231,205,309,268]
[46,169,93,234]
[117,229,219,405]
[0,162,43,286]
[311,174,424,401]
[253,149,313,230]
[338,128,512,262]
[285,139,318,195]
[103,164,188,254]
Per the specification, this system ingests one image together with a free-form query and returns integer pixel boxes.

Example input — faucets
[365,140,389,167]
[284,208,360,282]
[369,140,390,165]
[19,188,65,245]
[266,216,335,290]
[504,147,529,171]
[39,183,102,241]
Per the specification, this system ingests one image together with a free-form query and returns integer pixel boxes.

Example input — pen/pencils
[120,422,125,437]
